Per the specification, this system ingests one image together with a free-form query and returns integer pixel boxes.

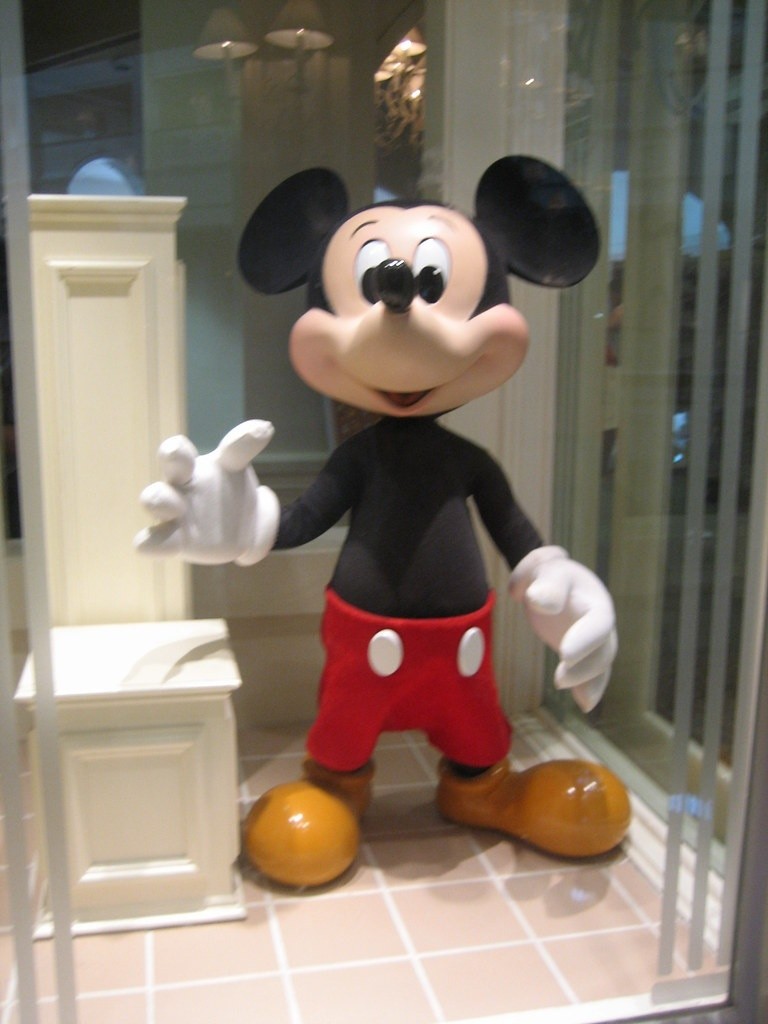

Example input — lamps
[263,0,336,89]
[191,8,259,61]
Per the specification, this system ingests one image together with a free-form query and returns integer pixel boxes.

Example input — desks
[14,616,245,941]
